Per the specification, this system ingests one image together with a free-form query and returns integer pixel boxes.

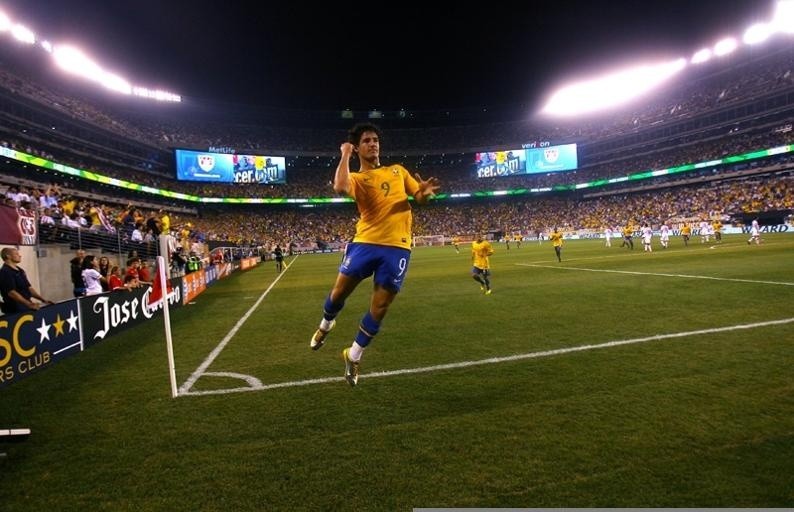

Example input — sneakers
[480,280,485,291]
[310,320,336,350]
[485,289,491,295]
[343,347,360,387]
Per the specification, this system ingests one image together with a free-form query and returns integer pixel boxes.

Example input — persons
[309,123,441,387]
